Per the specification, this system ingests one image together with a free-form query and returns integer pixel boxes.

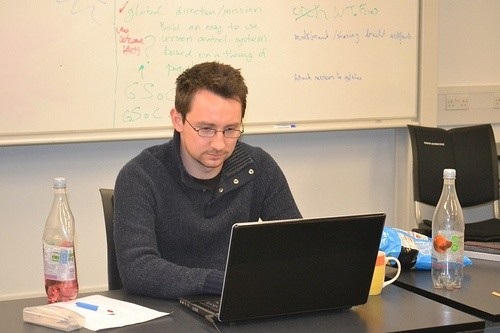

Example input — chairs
[407,123,500,247]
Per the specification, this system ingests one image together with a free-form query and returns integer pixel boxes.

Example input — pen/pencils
[76,302,114,315]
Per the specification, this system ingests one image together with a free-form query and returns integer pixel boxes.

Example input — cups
[368,251,402,295]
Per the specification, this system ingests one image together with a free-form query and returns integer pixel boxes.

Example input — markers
[273,124,296,128]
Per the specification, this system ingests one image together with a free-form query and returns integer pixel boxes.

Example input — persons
[114,62,302,296]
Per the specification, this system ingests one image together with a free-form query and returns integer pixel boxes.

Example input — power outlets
[495,93,500,109]
[446,95,469,110]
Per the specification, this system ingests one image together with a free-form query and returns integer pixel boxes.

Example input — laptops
[178,214,386,324]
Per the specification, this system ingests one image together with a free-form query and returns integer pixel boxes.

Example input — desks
[0,258,500,333]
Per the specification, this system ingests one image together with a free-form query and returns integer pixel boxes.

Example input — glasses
[181,113,245,138]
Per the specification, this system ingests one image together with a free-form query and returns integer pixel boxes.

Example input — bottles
[431,169,465,290]
[42,177,78,302]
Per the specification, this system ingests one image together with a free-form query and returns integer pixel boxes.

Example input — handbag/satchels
[379,225,472,270]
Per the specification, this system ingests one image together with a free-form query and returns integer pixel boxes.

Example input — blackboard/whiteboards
[0,0,423,146]
